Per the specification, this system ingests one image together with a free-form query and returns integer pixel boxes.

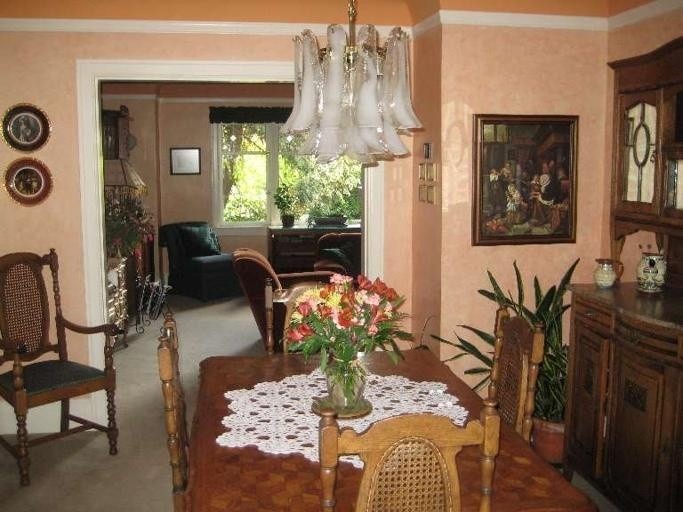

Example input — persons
[18,116,32,142]
[488,158,560,227]
[19,173,40,193]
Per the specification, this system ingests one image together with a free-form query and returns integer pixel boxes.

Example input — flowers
[277,274,414,401]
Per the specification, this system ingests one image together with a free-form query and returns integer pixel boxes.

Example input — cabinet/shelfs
[560,282,681,510]
[608,28,680,293]
[266,228,362,272]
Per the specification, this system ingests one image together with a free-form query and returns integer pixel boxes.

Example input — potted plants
[429,257,582,464]
[305,168,361,227]
[267,175,303,227]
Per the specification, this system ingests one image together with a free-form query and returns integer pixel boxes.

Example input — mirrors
[169,146,201,175]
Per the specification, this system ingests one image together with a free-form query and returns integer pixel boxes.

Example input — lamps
[277,0,422,163]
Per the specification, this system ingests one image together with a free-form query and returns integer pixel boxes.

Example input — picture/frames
[471,111,578,247]
[0,101,51,153]
[3,157,53,206]
[418,142,436,206]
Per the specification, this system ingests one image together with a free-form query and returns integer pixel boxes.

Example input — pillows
[181,224,221,257]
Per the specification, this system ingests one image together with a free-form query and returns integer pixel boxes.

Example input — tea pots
[591,257,625,289]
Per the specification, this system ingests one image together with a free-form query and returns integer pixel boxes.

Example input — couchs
[234,246,345,353]
[314,233,361,275]
[158,220,250,303]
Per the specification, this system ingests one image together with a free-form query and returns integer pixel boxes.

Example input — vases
[634,248,669,295]
[320,357,369,412]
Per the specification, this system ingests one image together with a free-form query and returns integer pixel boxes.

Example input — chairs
[317,400,503,511]
[155,307,192,511]
[0,249,127,488]
[481,306,548,444]
